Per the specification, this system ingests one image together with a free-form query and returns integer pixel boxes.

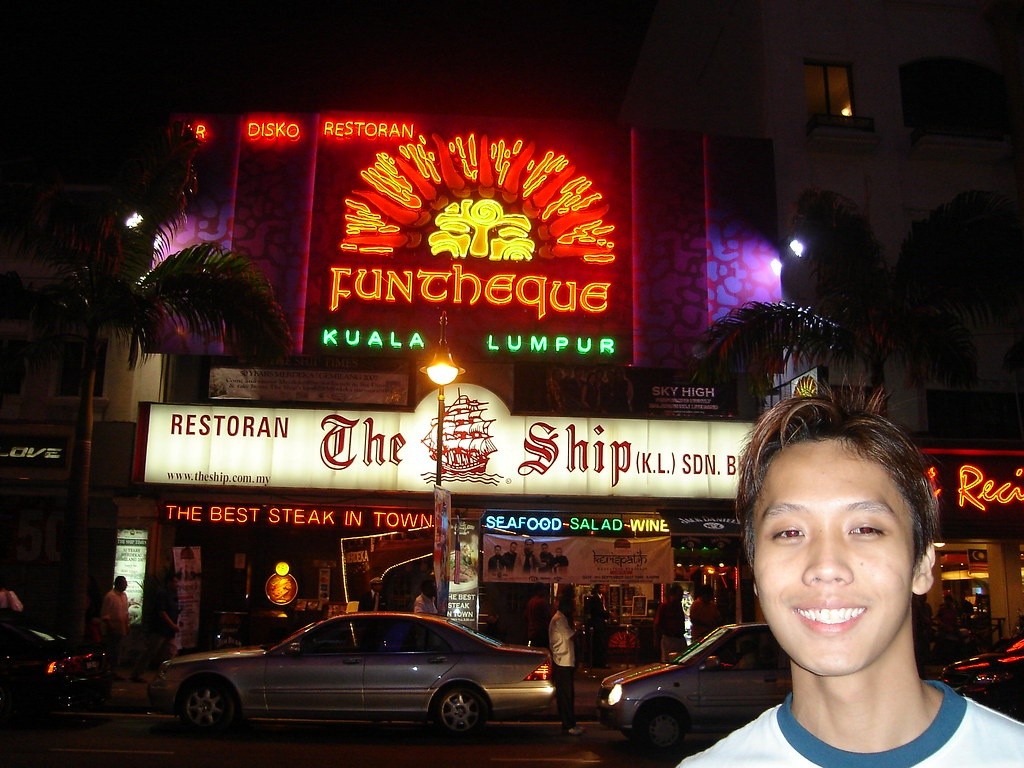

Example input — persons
[674,367,1024,768]
[0,585,23,613]
[687,585,723,644]
[558,584,581,672]
[909,589,971,675]
[652,585,687,660]
[131,571,181,683]
[99,576,128,681]
[357,577,391,613]
[587,584,611,668]
[523,582,551,647]
[548,596,587,737]
[413,579,438,615]
[488,538,569,576]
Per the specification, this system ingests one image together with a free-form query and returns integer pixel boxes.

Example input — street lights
[419,308,465,618]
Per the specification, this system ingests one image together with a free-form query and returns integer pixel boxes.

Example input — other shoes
[111,672,126,682]
[127,676,147,684]
[562,726,586,735]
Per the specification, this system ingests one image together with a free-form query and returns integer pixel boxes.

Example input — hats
[370,577,384,584]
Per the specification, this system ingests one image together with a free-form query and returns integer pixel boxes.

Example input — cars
[941,636,1024,722]
[147,611,603,739]
[596,622,792,757]
[0,611,113,725]
